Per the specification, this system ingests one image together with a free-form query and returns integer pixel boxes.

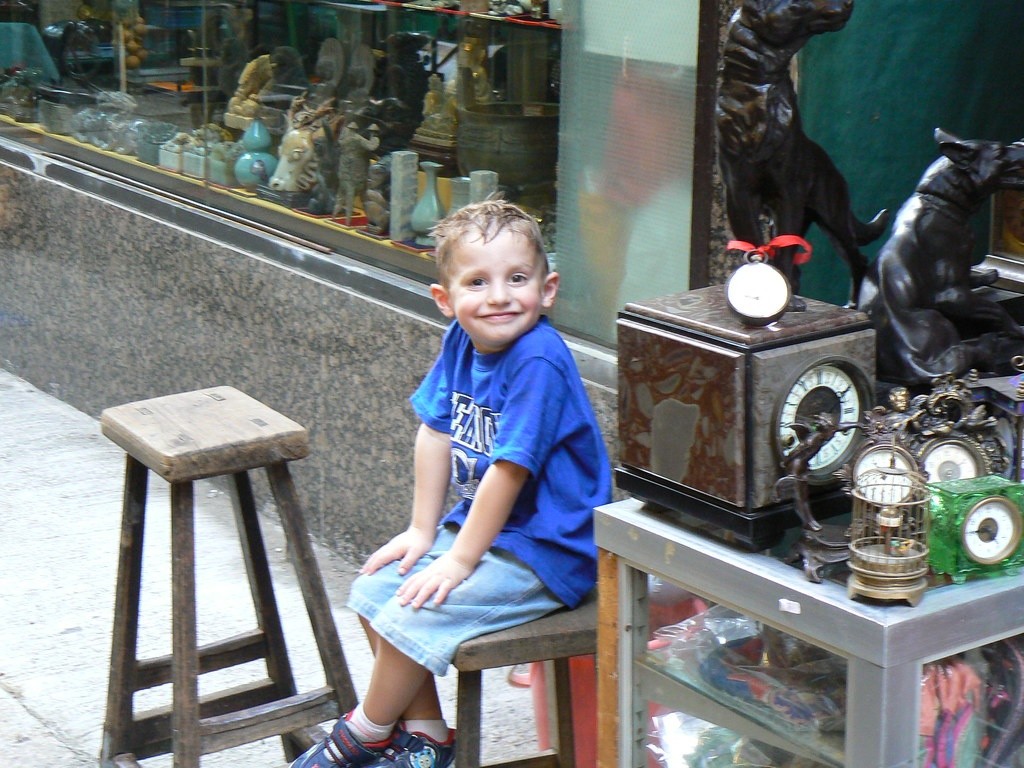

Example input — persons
[291,188,612,768]
[423,36,494,133]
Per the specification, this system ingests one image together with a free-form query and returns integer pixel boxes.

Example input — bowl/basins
[456,101,559,187]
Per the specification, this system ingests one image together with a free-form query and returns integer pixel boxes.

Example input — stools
[450,591,597,768]
[101,384,358,768]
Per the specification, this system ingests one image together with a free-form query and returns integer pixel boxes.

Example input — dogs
[715,0,1023,392]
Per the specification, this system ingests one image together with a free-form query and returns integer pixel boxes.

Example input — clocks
[615,234,1024,608]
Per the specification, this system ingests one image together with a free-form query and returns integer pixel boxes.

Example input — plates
[350,43,375,96]
[317,37,345,86]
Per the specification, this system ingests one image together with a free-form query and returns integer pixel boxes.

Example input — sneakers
[359,718,458,768]
[288,710,393,768]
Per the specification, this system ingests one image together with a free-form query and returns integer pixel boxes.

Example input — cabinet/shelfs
[593,497,1024,768]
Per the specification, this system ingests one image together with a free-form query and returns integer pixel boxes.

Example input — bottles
[411,160,446,246]
